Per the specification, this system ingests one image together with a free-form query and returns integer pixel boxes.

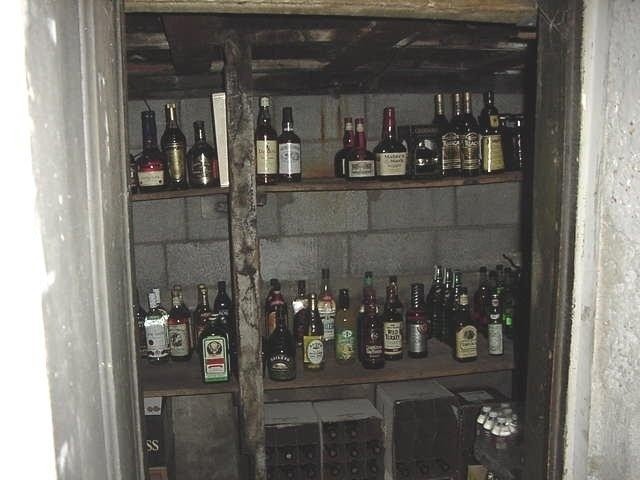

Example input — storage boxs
[263,379,461,479]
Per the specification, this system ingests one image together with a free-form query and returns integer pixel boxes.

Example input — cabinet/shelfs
[117,1,557,479]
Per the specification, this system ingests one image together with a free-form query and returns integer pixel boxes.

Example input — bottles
[454,287,479,362]
[451,93,463,177]
[214,281,233,326]
[364,270,375,302]
[187,120,219,188]
[291,280,309,348]
[474,403,519,465]
[267,304,296,381]
[380,285,404,361]
[142,292,170,364]
[345,118,376,181]
[254,95,278,186]
[334,289,359,365]
[389,276,403,314]
[497,112,509,142]
[136,111,166,192]
[278,107,302,184]
[427,265,444,337]
[175,286,195,349]
[196,289,214,352]
[461,92,479,177]
[432,93,451,178]
[502,268,515,340]
[452,272,464,305]
[439,268,453,342]
[516,270,527,320]
[267,283,287,337]
[167,291,192,362]
[498,264,504,296]
[507,115,529,170]
[359,288,383,369]
[264,278,279,329]
[335,117,354,178]
[482,91,504,175]
[419,283,433,340]
[302,293,326,370]
[432,262,441,292]
[198,312,231,384]
[152,289,170,321]
[485,271,506,336]
[487,294,504,356]
[160,103,187,187]
[409,283,428,358]
[375,108,407,181]
[133,287,146,352]
[475,267,488,328]
[195,284,206,329]
[317,269,336,343]
